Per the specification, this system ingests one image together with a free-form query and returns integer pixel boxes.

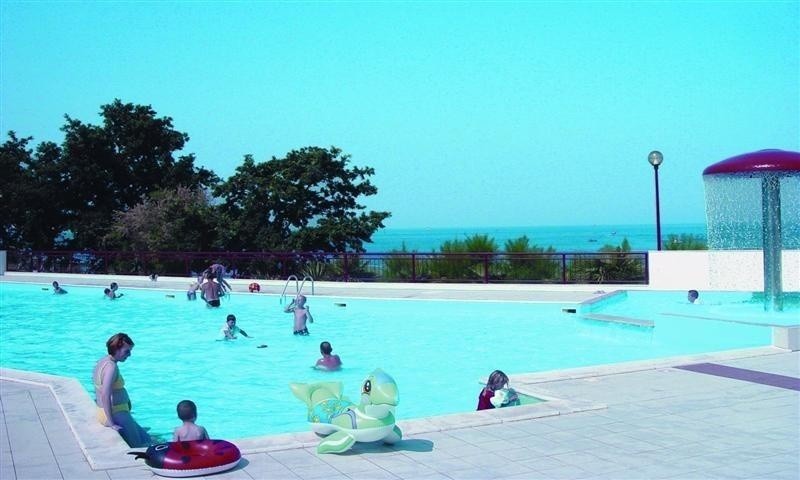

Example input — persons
[92,332,152,448]
[220,314,255,341]
[174,400,210,442]
[200,273,226,310]
[186,281,201,301]
[108,282,118,300]
[282,294,313,336]
[687,289,698,303]
[53,281,69,294]
[310,342,342,373]
[104,288,125,300]
[476,370,517,410]
[197,263,233,291]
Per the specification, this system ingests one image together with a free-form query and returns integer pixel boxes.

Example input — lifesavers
[289,369,402,453]
[127,440,241,477]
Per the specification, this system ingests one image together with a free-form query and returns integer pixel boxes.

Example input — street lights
[648,151,663,251]
